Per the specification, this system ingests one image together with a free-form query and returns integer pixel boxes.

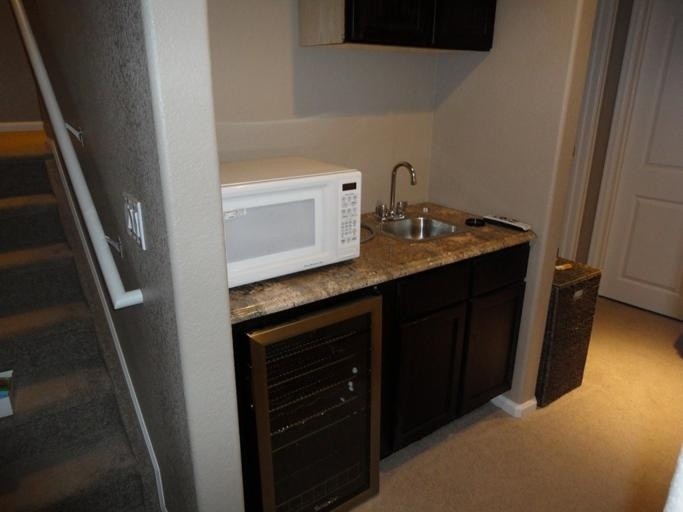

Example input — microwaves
[219,156,363,291]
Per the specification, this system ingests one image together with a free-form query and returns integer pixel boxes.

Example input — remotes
[482,213,530,232]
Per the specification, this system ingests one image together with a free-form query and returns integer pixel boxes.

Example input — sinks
[378,218,456,239]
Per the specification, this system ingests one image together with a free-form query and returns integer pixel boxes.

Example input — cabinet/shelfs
[381,241,530,461]
[295,0,497,52]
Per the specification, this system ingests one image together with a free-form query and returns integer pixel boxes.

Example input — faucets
[390,161,417,215]
